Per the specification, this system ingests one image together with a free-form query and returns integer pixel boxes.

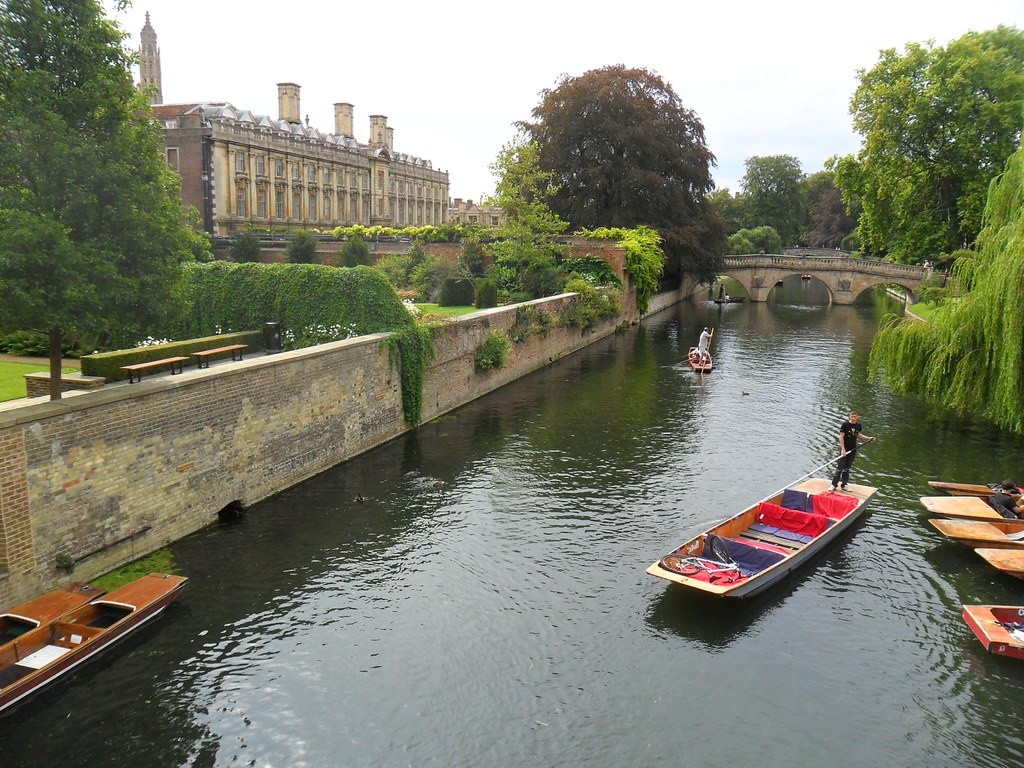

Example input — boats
[962,604,1024,658]
[0,573,190,713]
[919,481,1024,580]
[0,581,107,661]
[714,297,746,303]
[647,478,879,601]
[689,347,712,371]
[775,281,784,286]
[801,274,811,281]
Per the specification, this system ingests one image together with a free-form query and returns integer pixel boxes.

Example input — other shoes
[828,485,836,491]
[842,485,852,492]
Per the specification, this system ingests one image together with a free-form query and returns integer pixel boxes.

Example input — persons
[698,327,712,366]
[719,284,724,300]
[995,479,1024,519]
[726,293,729,300]
[827,410,876,493]
[923,259,933,278]
